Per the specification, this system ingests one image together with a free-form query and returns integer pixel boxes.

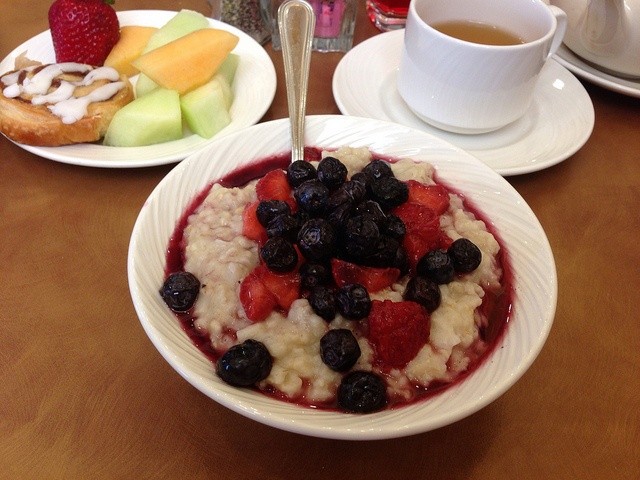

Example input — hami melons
[128,28,239,94]
[101,24,157,78]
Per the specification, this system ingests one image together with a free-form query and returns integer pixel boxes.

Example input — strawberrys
[48,0,120,66]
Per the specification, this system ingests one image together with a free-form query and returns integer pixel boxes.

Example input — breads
[0,66,134,146]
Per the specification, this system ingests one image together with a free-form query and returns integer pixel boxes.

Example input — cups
[399,0,567,135]
[366,1,411,33]
[203,0,272,46]
[258,0,361,52]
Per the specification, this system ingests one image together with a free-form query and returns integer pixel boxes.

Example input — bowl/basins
[128,114,559,441]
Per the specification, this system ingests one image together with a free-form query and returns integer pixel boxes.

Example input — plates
[551,0,640,98]
[332,27,596,176]
[0,9,277,169]
